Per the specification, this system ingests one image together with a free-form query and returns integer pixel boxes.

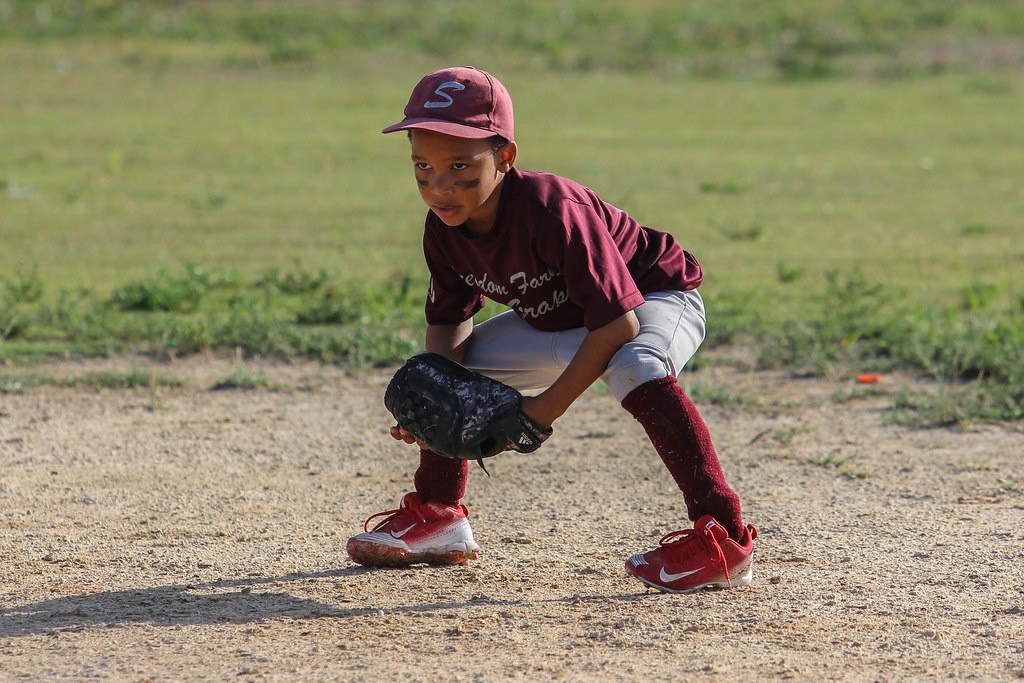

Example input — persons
[346,64,757,593]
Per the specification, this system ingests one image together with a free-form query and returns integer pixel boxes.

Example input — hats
[382,64,515,143]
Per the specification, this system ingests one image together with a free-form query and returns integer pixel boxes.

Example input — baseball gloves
[383,351,554,478]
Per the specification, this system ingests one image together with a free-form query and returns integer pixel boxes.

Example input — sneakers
[347,492,480,564]
[625,514,758,593]
